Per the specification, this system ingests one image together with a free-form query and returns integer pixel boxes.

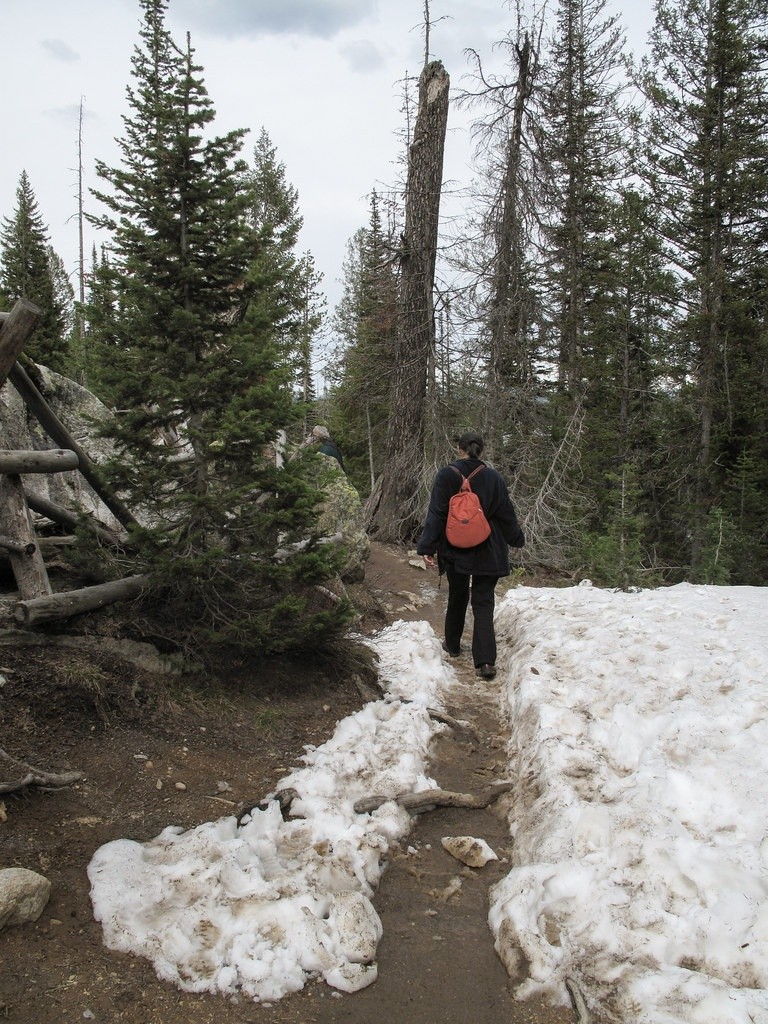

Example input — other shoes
[442,640,460,657]
[480,664,496,676]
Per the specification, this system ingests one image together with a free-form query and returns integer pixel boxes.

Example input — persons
[313,425,346,473]
[417,433,525,676]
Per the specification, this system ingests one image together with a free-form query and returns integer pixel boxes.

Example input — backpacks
[446,465,491,548]
